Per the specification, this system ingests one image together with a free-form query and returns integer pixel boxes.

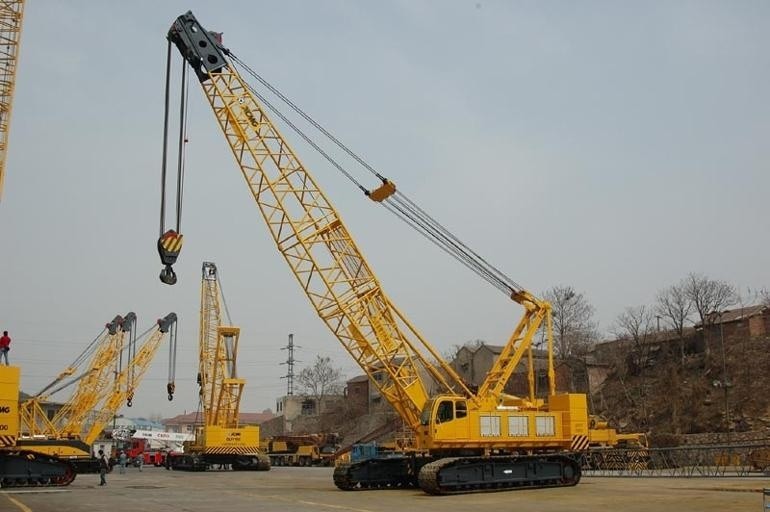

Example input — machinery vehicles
[0,312,180,491]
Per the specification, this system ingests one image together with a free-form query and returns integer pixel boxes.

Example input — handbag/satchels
[6,346,10,351]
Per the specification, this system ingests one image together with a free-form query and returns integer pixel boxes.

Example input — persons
[108,450,145,475]
[99,449,108,486]
[0,331,11,366]
